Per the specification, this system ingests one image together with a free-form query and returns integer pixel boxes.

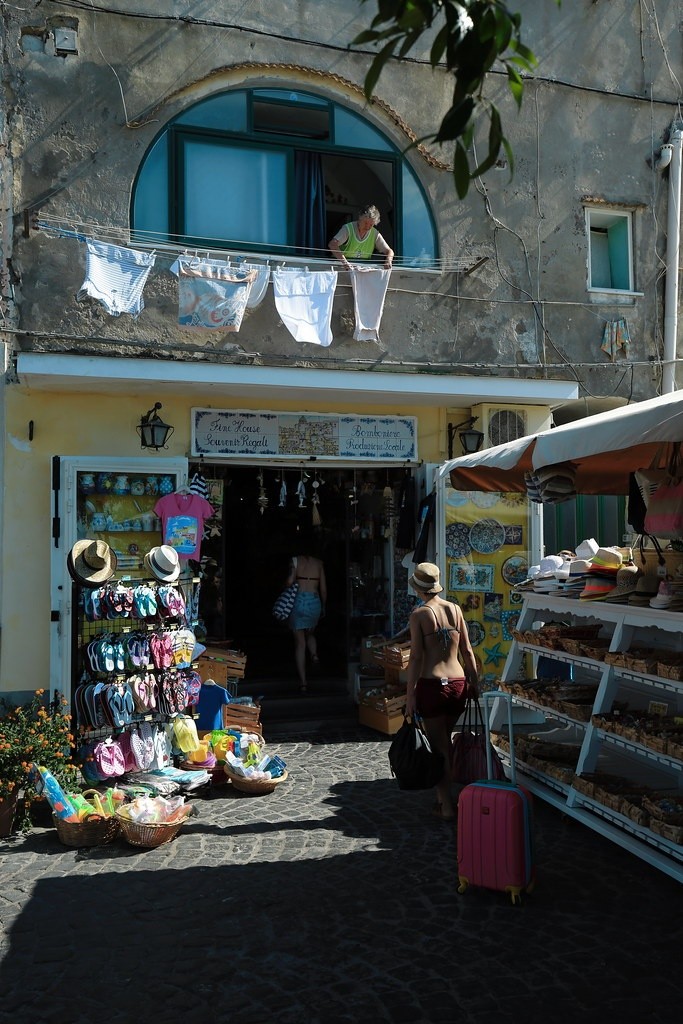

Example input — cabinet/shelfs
[488,593,683,884]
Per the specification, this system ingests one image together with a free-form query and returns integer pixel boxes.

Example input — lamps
[136,402,174,451]
[448,416,484,459]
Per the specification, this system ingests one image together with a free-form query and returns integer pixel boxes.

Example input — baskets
[115,802,189,847]
[224,761,288,792]
[52,789,120,847]
[490,622,682,844]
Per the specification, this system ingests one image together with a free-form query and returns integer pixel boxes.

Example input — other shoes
[311,654,319,670]
[299,684,307,693]
[432,803,452,822]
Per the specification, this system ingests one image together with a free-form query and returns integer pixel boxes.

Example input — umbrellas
[432,388,683,496]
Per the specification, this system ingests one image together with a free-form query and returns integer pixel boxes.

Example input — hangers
[203,669,214,686]
[174,475,194,495]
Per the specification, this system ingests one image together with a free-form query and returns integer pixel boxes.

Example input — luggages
[455,689,529,904]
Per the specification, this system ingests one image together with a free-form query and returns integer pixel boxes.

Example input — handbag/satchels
[387,713,438,791]
[271,579,299,621]
[448,695,505,785]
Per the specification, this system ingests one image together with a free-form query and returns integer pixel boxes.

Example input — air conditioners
[472,402,552,451]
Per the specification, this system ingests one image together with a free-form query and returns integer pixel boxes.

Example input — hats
[514,538,682,611]
[143,545,180,585]
[407,562,443,593]
[66,539,118,582]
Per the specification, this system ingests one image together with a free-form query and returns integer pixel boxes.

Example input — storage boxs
[347,636,412,734]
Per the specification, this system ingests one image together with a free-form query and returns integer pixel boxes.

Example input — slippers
[75,582,200,784]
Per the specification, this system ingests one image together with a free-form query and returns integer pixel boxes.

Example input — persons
[404,563,480,820]
[286,533,328,692]
[328,204,394,269]
